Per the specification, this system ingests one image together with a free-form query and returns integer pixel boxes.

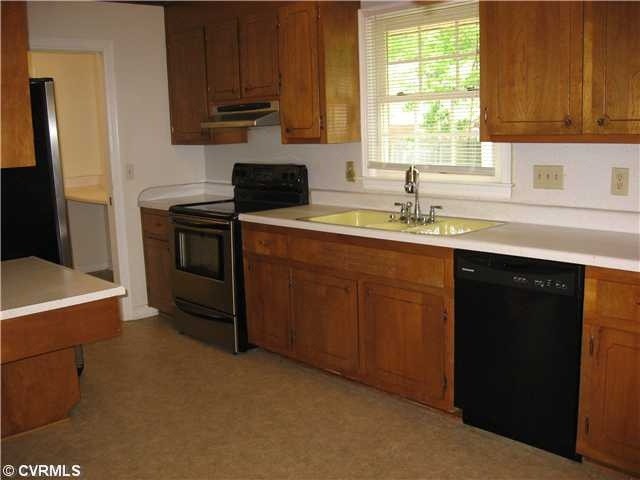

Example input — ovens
[167,200,242,357]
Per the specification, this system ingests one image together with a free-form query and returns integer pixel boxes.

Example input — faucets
[394,164,442,225]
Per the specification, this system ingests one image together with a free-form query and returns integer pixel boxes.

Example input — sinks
[307,210,402,227]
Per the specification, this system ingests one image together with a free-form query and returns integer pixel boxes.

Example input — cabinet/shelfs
[163,2,248,145]
[277,1,361,144]
[140,205,172,321]
[205,2,278,114]
[2,2,36,170]
[576,258,640,478]
[480,1,640,143]
[241,217,289,358]
[290,227,452,412]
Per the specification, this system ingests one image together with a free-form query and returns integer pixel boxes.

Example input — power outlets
[532,165,565,188]
[611,168,630,197]
